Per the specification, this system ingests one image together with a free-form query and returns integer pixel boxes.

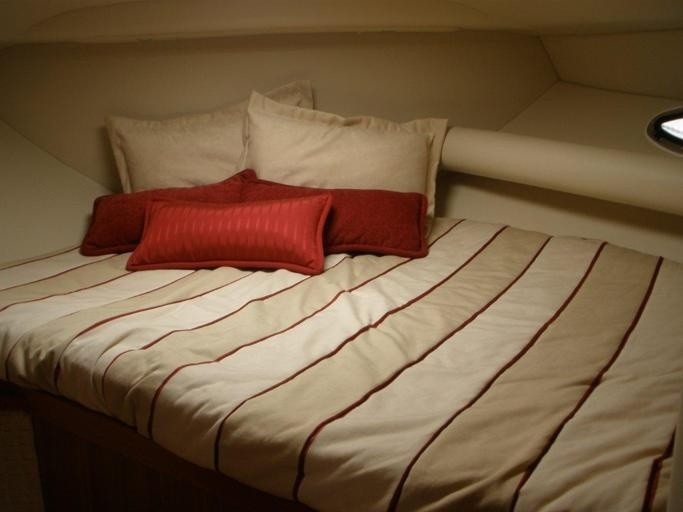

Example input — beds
[0,209,683,511]
